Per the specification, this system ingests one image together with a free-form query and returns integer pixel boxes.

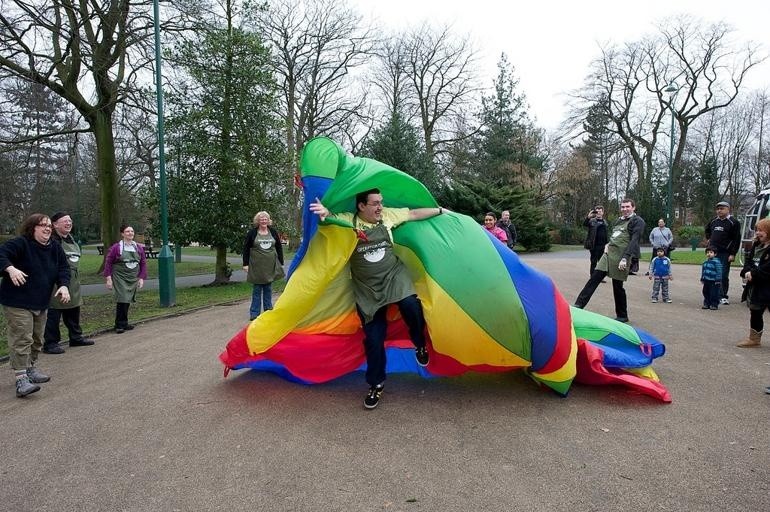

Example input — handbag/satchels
[669,245,675,250]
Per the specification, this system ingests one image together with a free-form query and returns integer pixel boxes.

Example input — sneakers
[652,298,673,303]
[30,371,50,383]
[615,317,629,322]
[720,298,729,305]
[702,305,718,310]
[116,328,124,333]
[414,345,430,367]
[16,379,41,397]
[43,342,65,354]
[364,382,384,408]
[124,325,134,330]
[70,337,94,346]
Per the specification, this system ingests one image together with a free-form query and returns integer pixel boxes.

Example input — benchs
[97,246,103,255]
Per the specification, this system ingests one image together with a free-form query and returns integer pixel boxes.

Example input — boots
[738,328,763,348]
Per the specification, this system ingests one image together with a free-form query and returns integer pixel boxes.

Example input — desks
[142,246,160,259]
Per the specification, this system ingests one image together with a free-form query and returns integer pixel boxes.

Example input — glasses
[367,202,384,207]
[37,223,52,228]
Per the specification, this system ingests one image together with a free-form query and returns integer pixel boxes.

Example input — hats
[717,202,730,208]
[51,210,71,221]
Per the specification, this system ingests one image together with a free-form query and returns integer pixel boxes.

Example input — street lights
[665,78,679,228]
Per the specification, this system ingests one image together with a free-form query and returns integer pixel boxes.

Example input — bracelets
[439,206,444,215]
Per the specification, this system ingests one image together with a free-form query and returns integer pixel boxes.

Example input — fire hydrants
[689,237,699,251]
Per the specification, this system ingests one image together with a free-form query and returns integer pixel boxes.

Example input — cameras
[592,211,598,214]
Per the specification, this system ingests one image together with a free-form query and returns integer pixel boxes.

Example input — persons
[481,212,511,248]
[584,206,609,284]
[308,189,451,409]
[244,211,285,321]
[1,212,71,396]
[103,224,146,334]
[649,216,675,262]
[647,247,673,304]
[573,199,647,325]
[700,246,722,310]
[737,217,770,350]
[704,202,742,304]
[498,211,517,251]
[39,210,96,353]
